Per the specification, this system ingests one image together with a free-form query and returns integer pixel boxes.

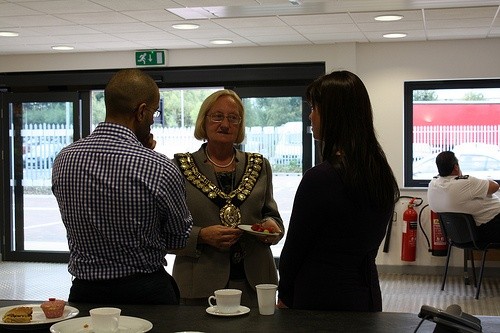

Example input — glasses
[205,112,243,125]
[137,103,161,118]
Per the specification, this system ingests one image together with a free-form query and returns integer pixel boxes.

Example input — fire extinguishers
[431,208,447,257]
[401,198,418,262]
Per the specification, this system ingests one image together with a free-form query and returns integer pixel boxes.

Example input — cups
[90,308,121,333]
[208,289,242,313]
[256,284,278,315]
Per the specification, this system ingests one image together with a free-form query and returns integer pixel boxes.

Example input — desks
[0,299,500,333]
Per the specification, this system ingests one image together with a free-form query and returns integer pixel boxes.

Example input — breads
[2,307,33,323]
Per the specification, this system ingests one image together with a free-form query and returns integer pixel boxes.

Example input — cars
[412,141,500,182]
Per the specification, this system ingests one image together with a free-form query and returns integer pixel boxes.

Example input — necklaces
[204,145,235,167]
[176,150,263,228]
[213,162,235,194]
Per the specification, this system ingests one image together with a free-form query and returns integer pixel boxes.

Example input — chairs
[436,212,500,300]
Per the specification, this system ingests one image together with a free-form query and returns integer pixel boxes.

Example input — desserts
[40,298,65,318]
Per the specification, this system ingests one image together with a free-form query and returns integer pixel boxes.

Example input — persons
[276,70,401,313]
[427,151,500,244]
[172,88,284,306]
[51,69,193,303]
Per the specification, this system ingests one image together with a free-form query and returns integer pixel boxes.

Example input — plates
[0,305,79,325]
[238,225,280,236]
[206,306,250,316]
[50,316,153,333]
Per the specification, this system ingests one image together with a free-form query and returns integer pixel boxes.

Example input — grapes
[251,224,274,233]
